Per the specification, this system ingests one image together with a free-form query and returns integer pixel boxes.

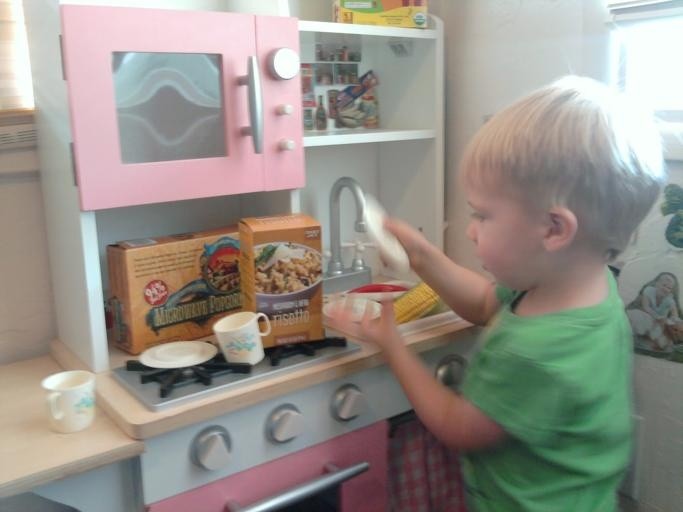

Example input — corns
[392,278,442,327]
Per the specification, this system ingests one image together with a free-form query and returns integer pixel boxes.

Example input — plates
[141,339,219,370]
[323,299,382,322]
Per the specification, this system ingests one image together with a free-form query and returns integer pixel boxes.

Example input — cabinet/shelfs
[0,0,477,512]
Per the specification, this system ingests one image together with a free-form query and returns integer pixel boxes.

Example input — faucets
[325,176,367,274]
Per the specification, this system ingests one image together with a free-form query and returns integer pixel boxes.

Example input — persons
[325,75,664,512]
[641,274,679,351]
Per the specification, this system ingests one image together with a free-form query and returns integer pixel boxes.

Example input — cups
[213,310,271,369]
[41,369,97,434]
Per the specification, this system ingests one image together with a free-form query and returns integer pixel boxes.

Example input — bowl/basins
[254,243,325,305]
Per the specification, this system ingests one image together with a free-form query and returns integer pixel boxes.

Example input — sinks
[322,279,464,339]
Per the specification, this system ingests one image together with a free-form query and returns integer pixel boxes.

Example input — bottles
[360,96,378,128]
[316,94,328,131]
[299,62,315,130]
